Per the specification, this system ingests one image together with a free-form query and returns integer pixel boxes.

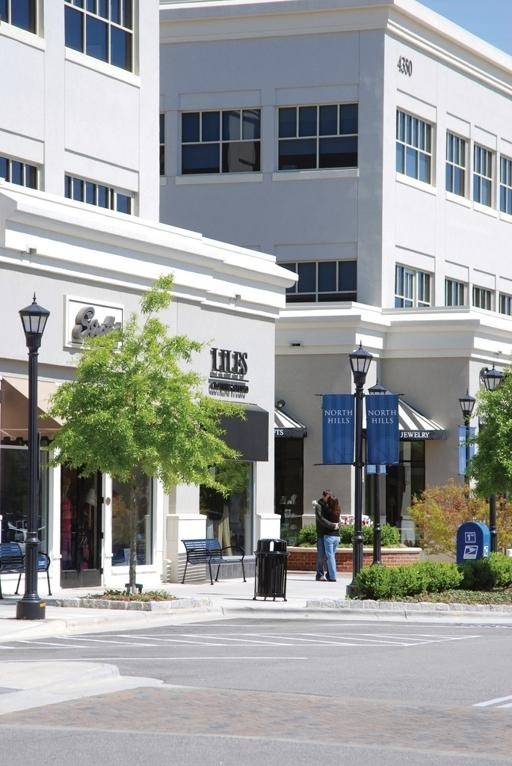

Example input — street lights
[15,291,50,601]
[348,340,387,583]
[458,362,505,551]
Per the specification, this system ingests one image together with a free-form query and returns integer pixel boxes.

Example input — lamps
[227,295,242,304]
[20,247,36,257]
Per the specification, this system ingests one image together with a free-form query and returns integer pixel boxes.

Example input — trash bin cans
[253,539,289,601]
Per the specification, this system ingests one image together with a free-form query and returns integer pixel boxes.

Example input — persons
[314,490,341,582]
[208,479,233,556]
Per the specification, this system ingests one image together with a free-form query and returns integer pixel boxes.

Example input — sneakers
[315,573,336,582]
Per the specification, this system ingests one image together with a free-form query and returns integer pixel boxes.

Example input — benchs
[0,543,51,600]
[179,538,246,586]
